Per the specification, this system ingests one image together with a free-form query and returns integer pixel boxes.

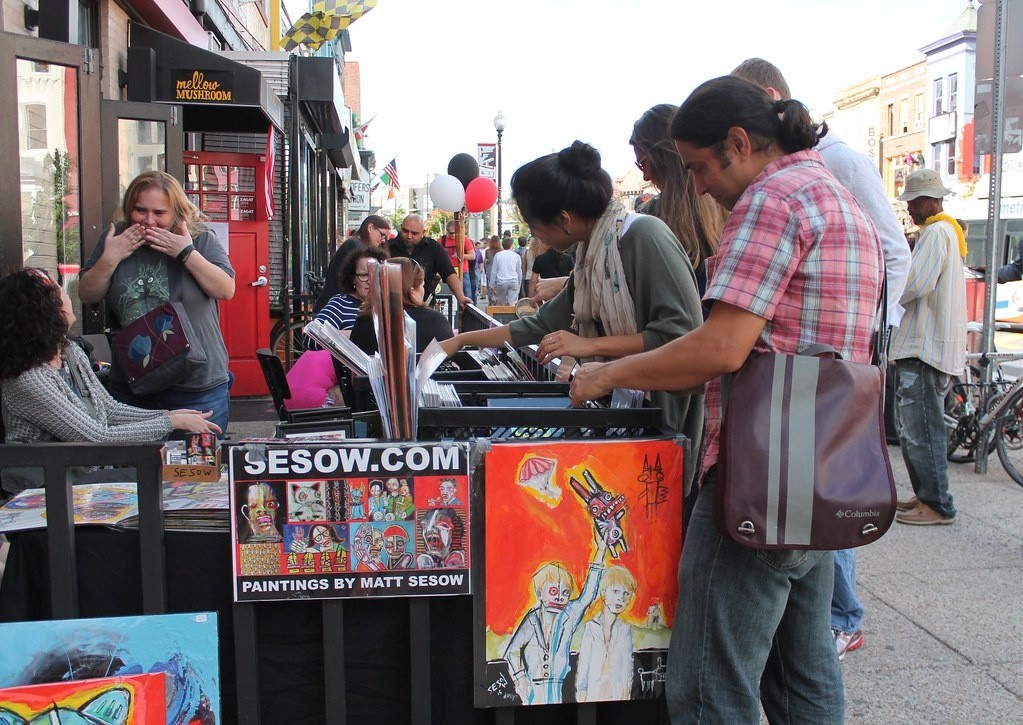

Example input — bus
[943,194,1023,333]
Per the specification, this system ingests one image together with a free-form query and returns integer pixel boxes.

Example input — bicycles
[944,321,1023,485]
[270,272,326,375]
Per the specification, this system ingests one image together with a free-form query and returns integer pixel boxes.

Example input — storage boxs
[160,445,222,483]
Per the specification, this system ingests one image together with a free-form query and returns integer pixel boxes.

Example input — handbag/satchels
[713,348,898,552]
[106,302,207,395]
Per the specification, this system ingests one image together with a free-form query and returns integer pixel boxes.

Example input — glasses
[354,272,370,282]
[412,258,420,272]
[448,230,455,234]
[374,226,387,240]
[635,156,647,172]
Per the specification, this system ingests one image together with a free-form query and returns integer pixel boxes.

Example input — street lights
[494,112,506,238]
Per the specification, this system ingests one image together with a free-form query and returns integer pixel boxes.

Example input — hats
[897,169,954,201]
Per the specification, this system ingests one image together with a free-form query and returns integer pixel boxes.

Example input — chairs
[330,353,355,412]
[233,596,432,725]
[0,441,166,614]
[256,348,353,423]
[79,334,112,373]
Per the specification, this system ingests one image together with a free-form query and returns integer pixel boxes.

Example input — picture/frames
[276,418,355,441]
[433,294,452,330]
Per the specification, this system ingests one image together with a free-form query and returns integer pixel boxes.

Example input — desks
[353,381,630,441]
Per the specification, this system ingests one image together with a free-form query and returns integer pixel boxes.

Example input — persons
[0,171,235,498]
[283,349,346,410]
[440,141,707,494]
[975,235,1023,285]
[302,251,454,410]
[894,169,967,526]
[469,221,575,307]
[629,57,911,725]
[310,214,473,321]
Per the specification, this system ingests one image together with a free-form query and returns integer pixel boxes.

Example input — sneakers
[896,502,956,524]
[896,496,919,510]
[832,628,863,661]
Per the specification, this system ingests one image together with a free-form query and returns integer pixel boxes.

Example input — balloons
[429,175,465,212]
[466,176,498,213]
[448,153,479,189]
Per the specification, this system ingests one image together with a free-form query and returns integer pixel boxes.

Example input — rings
[549,337,554,344]
[569,390,572,397]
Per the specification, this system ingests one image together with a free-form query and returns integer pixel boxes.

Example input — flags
[278,0,377,51]
[380,158,400,199]
[265,127,276,221]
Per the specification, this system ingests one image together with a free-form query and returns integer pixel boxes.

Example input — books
[0,473,232,532]
[303,262,535,440]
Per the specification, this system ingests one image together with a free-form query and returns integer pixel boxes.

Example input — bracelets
[562,278,569,288]
[177,244,196,263]
